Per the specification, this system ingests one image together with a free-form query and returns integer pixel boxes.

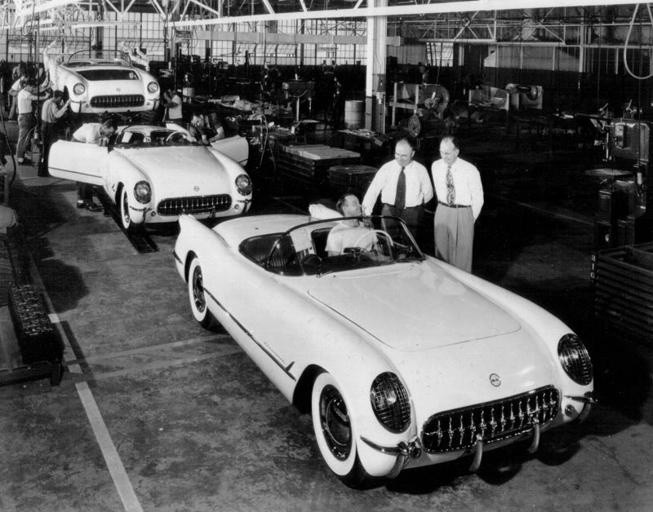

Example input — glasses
[394,151,411,160]
[440,150,456,157]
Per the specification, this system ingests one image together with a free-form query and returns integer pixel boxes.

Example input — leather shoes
[84,202,102,212]
[17,157,33,166]
[76,200,85,208]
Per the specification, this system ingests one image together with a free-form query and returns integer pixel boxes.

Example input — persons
[432,134,484,273]
[70,120,116,213]
[1,67,9,111]
[325,194,380,256]
[36,91,71,178]
[189,112,233,146]
[11,61,46,81]
[161,90,183,132]
[8,74,24,121]
[16,81,50,171]
[360,139,432,253]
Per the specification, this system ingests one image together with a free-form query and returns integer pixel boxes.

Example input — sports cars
[43,48,160,113]
[48,124,252,230]
[173,204,598,489]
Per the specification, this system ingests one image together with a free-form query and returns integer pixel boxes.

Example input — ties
[393,165,406,218]
[445,165,456,208]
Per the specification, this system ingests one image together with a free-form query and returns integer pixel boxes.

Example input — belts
[382,202,422,211]
[435,200,472,209]
